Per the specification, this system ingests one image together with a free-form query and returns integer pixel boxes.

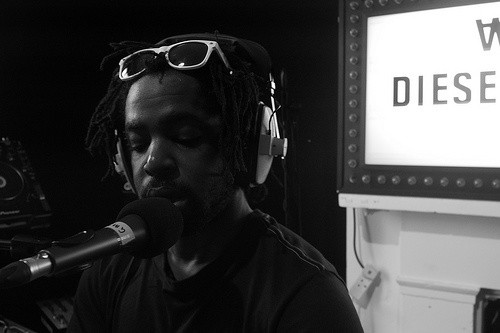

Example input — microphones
[0,196,185,287]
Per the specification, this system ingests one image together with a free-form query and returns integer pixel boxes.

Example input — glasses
[117,38,234,82]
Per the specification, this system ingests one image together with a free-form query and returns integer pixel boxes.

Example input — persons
[63,33,363,333]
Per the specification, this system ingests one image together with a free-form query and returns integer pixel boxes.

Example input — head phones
[111,33,288,195]
